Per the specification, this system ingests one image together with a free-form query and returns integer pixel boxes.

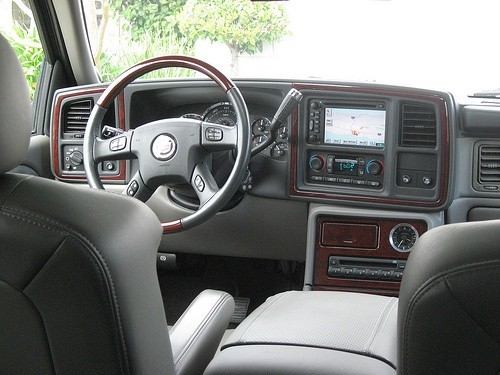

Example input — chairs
[397,217,499,375]
[0,33,237,375]
